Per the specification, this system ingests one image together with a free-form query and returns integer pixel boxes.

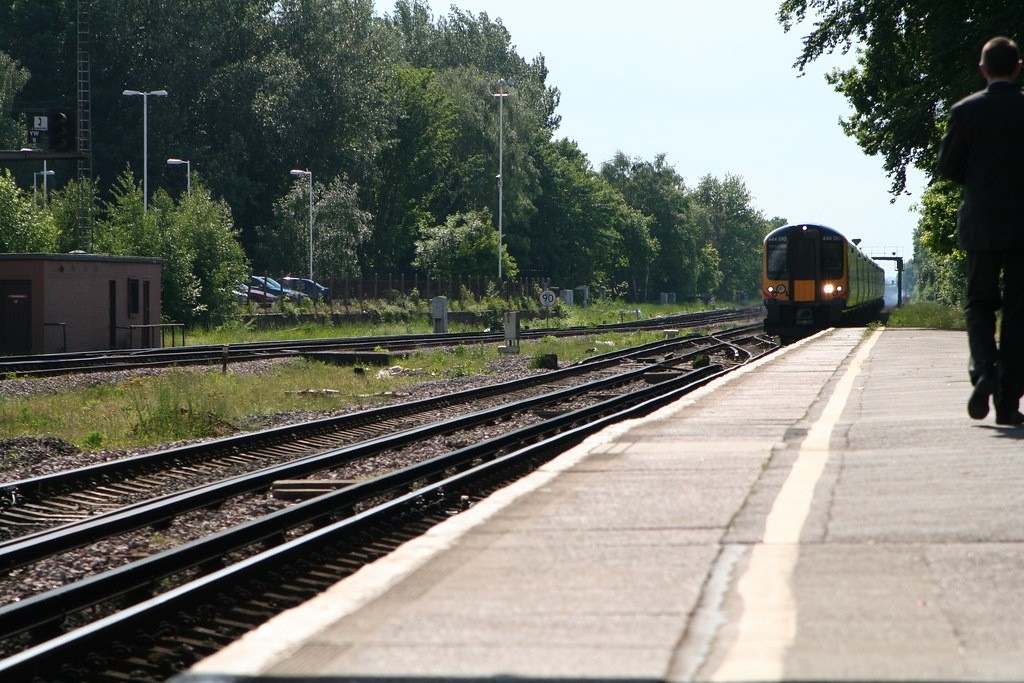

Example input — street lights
[491,77,512,276]
[290,169,313,280]
[123,89,169,216]
[33,170,56,208]
[167,158,191,198]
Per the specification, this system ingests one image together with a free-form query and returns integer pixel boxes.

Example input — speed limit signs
[539,290,557,308]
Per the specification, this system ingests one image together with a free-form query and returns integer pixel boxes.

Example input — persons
[936,36,1024,425]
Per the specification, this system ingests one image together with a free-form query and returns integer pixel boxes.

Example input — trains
[760,222,887,336]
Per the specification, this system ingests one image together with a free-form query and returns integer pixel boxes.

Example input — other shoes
[968,374,993,420]
[996,410,1024,425]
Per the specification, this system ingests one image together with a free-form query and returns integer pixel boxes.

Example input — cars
[276,277,331,305]
[222,276,309,310]
